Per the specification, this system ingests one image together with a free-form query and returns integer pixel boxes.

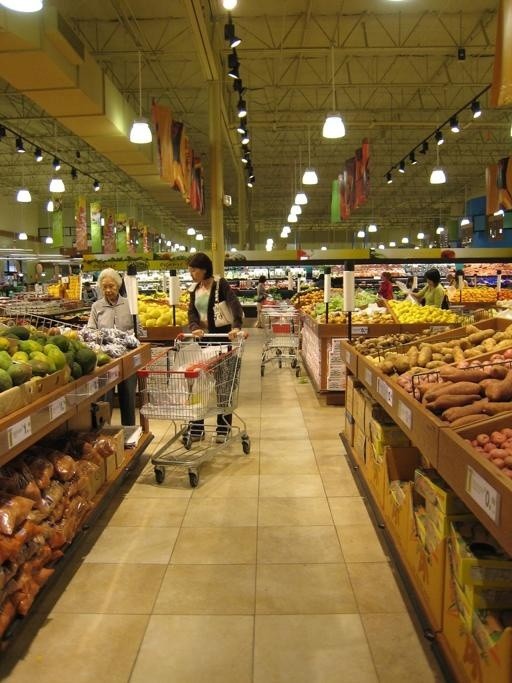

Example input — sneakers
[215,435,230,443]
[179,432,205,443]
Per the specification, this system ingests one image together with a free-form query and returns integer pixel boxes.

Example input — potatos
[365,324,512,375]
[418,364,512,429]
[350,329,461,355]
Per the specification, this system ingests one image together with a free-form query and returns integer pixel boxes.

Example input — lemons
[389,299,462,324]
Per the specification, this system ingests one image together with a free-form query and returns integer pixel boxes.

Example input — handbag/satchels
[213,278,245,327]
[421,295,449,310]
[254,295,264,302]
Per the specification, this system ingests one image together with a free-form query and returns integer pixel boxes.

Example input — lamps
[129,51,153,144]
[265,43,346,252]
[0,123,101,244]
[223,12,256,189]
[159,228,203,253]
[357,99,505,253]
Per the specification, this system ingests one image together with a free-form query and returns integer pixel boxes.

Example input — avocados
[0,316,110,397]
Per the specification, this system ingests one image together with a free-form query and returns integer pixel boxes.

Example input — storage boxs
[95,426,125,492]
[91,401,111,429]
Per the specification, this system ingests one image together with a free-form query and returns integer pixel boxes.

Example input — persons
[314,274,336,288]
[378,272,393,300]
[86,267,147,426]
[253,275,272,328]
[446,274,456,287]
[179,253,245,443]
[82,282,97,303]
[450,269,468,288]
[405,267,449,310]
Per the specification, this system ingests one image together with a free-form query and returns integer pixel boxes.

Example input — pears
[138,301,188,327]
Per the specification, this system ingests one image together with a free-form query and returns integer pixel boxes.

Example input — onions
[463,429,512,482]
[397,349,512,401]
